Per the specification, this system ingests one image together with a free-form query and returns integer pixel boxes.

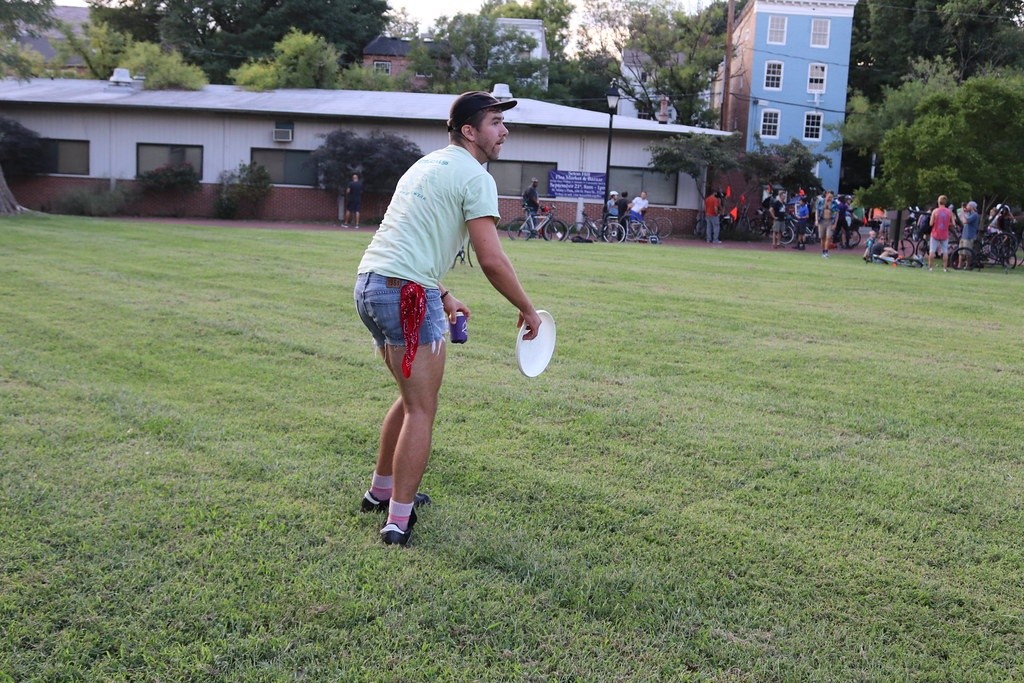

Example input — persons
[704,189,722,244]
[522,177,542,239]
[356,91,542,546]
[918,195,1016,272]
[607,190,648,243]
[762,188,858,259]
[863,206,902,264]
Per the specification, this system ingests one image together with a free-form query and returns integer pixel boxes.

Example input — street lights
[604,81,620,221]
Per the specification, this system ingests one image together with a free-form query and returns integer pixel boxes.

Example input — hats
[609,190,619,196]
[447,91,518,132]
[968,201,977,211]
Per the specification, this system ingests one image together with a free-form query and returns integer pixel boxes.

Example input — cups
[449,311,467,343]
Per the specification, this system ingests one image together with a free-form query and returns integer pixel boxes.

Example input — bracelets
[441,290,450,299]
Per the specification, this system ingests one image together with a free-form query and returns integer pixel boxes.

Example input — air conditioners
[272,129,292,141]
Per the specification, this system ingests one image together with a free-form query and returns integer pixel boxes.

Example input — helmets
[916,214,928,229]
[531,178,538,187]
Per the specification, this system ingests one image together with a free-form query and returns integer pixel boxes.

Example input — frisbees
[515,308,558,379]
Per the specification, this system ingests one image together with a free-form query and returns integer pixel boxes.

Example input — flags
[740,195,746,204]
[726,186,731,198]
[730,207,738,222]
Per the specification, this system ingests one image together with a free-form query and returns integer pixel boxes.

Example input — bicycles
[916,230,1024,270]
[507,203,569,242]
[567,209,626,243]
[883,223,915,257]
[748,207,861,248]
[591,211,674,244]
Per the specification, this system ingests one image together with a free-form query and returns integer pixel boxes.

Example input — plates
[516,310,556,378]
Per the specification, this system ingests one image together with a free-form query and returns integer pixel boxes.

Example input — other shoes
[713,240,722,243]
[707,240,712,243]
[340,223,348,228]
[929,268,933,271]
[794,241,806,250]
[943,268,948,271]
[379,506,418,546]
[822,250,829,258]
[354,225,359,229]
[360,491,431,512]
